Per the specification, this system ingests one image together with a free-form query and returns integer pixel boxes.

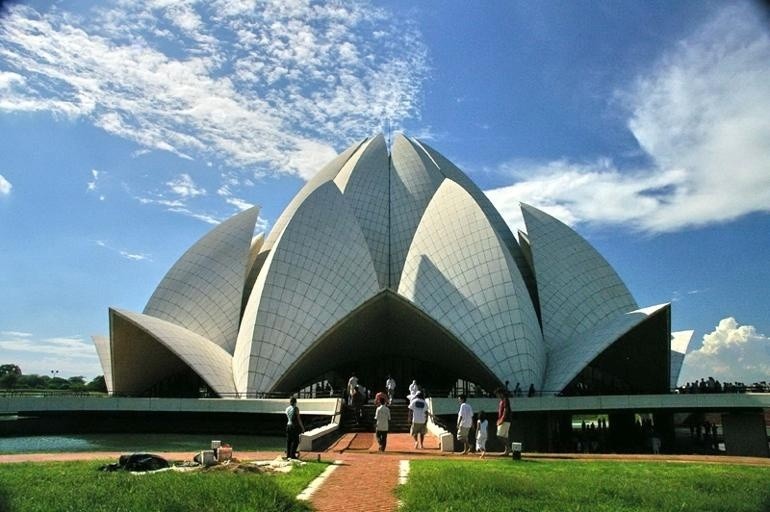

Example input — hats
[416,390,422,395]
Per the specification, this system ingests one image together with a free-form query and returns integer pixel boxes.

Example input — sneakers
[462,445,511,458]
[414,441,424,448]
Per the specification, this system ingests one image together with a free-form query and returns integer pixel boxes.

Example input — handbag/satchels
[288,406,299,423]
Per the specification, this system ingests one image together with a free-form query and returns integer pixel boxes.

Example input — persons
[574,416,720,455]
[678,376,770,395]
[455,392,473,457]
[406,391,430,451]
[475,409,489,460]
[284,396,305,459]
[317,372,537,427]
[494,387,514,457]
[373,396,392,454]
